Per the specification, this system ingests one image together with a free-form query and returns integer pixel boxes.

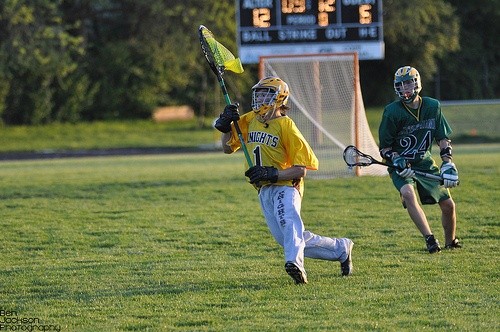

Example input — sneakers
[445,239,463,248]
[425,234,441,253]
[340,239,354,275]
[285,261,307,285]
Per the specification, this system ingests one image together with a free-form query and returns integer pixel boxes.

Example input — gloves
[439,161,458,188]
[245,164,278,185]
[214,103,241,133]
[389,153,415,177]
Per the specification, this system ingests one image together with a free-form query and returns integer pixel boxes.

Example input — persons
[378,66,462,253]
[215,77,355,284]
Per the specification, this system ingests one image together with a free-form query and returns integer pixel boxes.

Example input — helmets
[393,66,422,103]
[252,76,290,117]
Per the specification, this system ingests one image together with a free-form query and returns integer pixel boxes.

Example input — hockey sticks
[197,23,262,188]
[342,145,461,187]
